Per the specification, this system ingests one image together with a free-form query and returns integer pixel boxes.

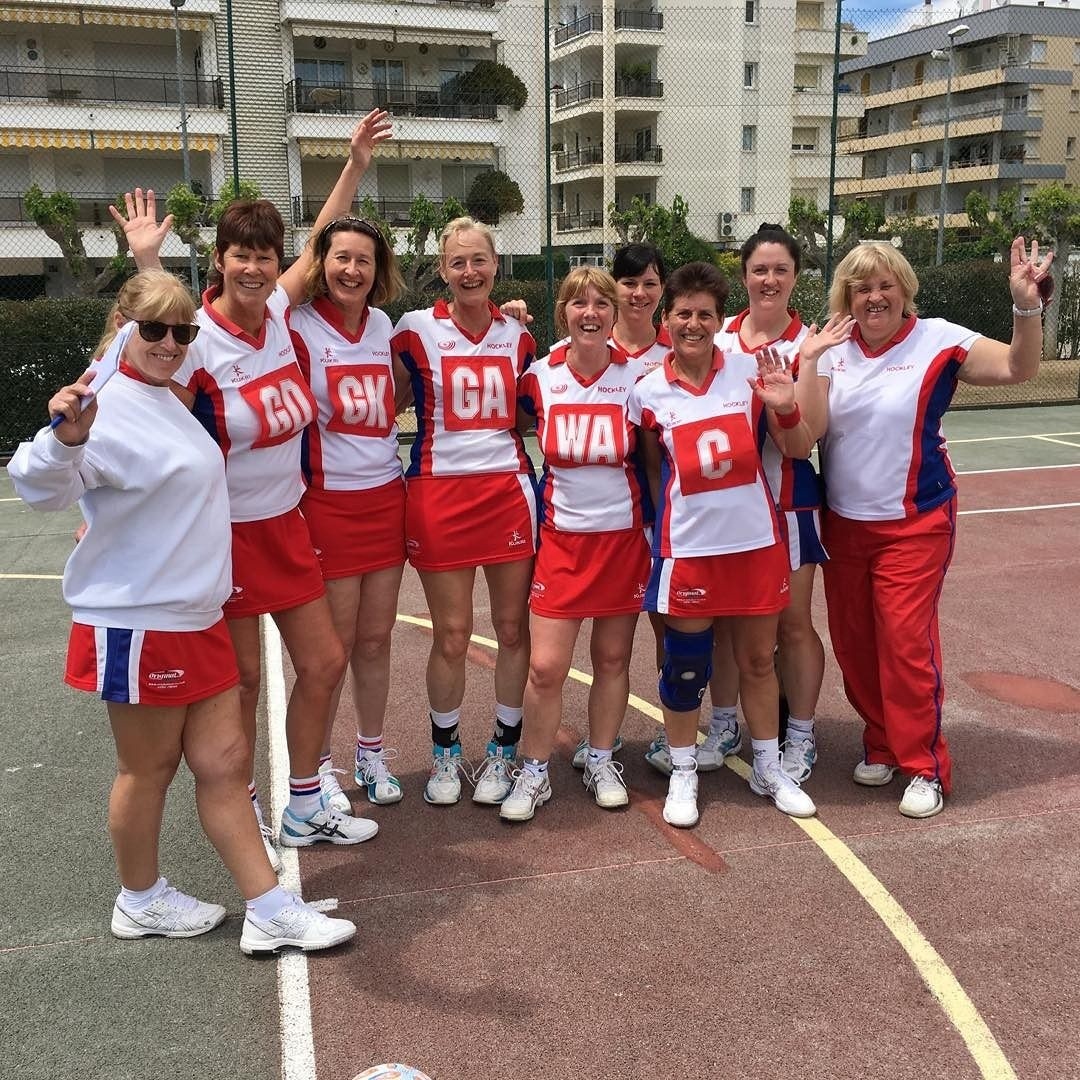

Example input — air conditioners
[718,212,738,236]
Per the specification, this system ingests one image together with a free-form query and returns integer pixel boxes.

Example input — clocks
[458,46,469,56]
[357,63,368,74]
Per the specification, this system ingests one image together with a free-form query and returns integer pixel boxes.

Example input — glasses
[119,312,201,345]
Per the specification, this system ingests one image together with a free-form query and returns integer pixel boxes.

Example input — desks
[48,89,81,98]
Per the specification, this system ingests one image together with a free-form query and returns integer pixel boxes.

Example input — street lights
[931,23,969,265]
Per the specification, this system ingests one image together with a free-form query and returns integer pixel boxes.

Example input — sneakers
[748,758,817,818]
[498,771,552,822]
[582,754,629,808]
[110,885,227,942]
[257,824,283,873]
[899,774,944,818]
[662,769,699,829]
[472,753,520,806]
[424,754,465,805]
[853,758,901,786]
[354,748,403,805]
[695,718,742,771]
[645,736,675,776]
[572,734,622,769]
[279,805,379,847]
[239,892,357,957]
[318,759,353,815]
[779,735,817,784]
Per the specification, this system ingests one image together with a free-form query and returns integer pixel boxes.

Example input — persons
[625,260,818,828]
[797,235,1056,820]
[498,264,649,822]
[77,104,395,853]
[7,274,360,959]
[500,242,679,773]
[107,185,402,813]
[709,222,827,785]
[387,211,537,806]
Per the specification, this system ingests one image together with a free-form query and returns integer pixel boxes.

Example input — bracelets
[776,402,801,429]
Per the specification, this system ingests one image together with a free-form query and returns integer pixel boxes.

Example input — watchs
[1012,296,1047,318]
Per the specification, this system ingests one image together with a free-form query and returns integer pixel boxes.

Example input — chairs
[309,89,348,115]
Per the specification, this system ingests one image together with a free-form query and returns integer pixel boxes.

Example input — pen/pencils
[44,415,66,435]
